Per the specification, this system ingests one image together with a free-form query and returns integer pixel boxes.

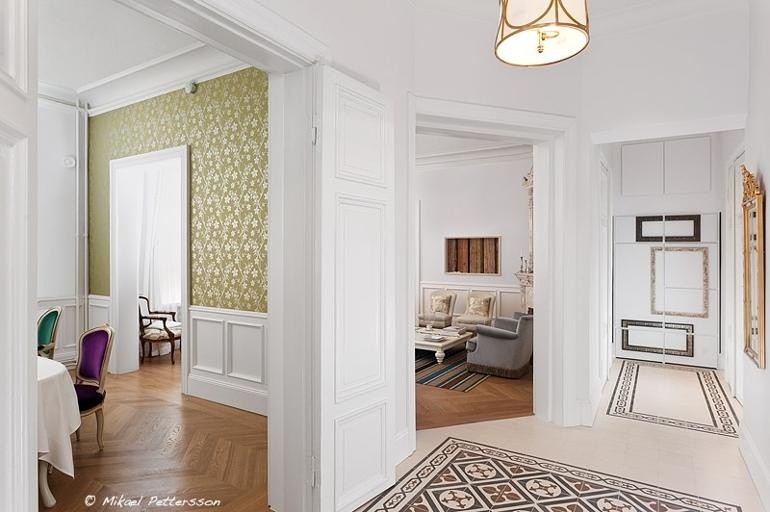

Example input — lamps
[494,0,588,67]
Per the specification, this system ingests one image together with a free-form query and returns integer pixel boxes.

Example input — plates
[424,337,444,342]
[418,328,441,333]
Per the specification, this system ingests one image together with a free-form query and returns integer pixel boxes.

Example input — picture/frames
[739,163,766,370]
[441,234,501,276]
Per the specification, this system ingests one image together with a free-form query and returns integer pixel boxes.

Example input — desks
[36,355,67,508]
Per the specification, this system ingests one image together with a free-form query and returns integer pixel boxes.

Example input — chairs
[139,295,182,366]
[36,306,116,451]
[418,289,495,343]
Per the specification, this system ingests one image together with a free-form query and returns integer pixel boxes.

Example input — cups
[426,324,432,331]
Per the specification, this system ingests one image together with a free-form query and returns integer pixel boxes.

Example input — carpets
[415,347,490,393]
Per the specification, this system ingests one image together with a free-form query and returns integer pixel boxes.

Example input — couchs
[466,312,533,379]
